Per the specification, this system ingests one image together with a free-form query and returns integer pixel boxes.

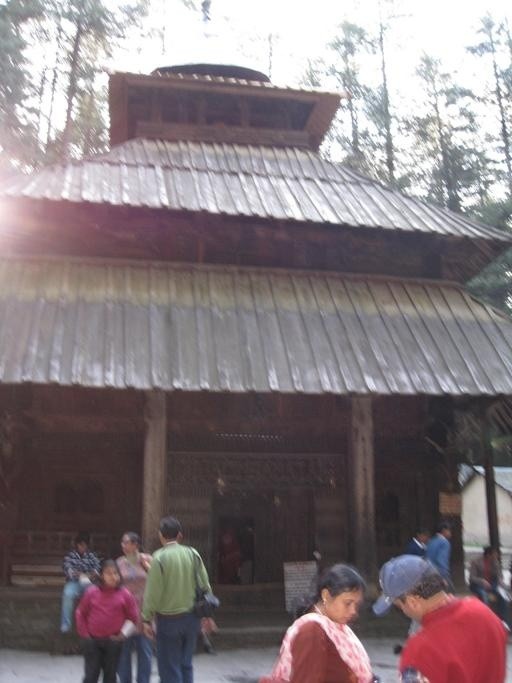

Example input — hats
[371,553,434,616]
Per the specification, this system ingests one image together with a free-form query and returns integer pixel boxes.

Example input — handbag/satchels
[193,588,218,618]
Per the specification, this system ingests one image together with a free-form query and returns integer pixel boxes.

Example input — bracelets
[143,620,151,624]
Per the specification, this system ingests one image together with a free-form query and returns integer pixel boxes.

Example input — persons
[113,531,154,683]
[139,514,222,682]
[258,561,376,682]
[425,521,456,596]
[371,552,511,683]
[469,545,511,635]
[60,533,104,633]
[403,527,430,557]
[74,557,144,683]
